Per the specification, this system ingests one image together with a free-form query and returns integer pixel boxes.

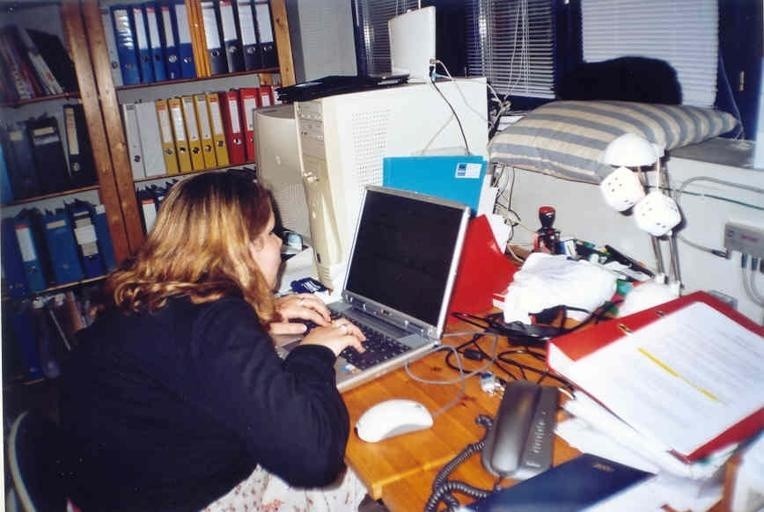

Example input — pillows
[488,99,737,187]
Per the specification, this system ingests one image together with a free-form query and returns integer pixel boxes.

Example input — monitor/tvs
[387,5,437,83]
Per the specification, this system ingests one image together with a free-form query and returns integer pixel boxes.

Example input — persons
[58,171,368,512]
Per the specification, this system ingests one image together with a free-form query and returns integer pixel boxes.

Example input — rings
[299,296,306,307]
[340,322,350,335]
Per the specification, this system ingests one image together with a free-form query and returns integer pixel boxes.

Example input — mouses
[354,398,434,444]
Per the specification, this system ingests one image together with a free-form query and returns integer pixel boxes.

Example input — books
[0,25,79,103]
[465,451,656,512]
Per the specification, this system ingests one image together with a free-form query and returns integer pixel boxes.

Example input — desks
[271,248,634,512]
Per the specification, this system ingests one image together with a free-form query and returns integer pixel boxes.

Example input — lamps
[597,134,688,288]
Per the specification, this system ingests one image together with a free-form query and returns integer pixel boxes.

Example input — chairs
[9,411,76,512]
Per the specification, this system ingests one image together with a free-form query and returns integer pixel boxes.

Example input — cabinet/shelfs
[86,1,291,259]
[2,0,124,415]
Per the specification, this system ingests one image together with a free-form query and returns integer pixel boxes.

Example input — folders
[120,85,282,179]
[96,0,279,88]
[0,199,116,299]
[6,99,94,199]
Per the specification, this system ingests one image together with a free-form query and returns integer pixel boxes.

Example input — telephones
[482,379,561,481]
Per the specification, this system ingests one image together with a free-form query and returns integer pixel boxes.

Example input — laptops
[267,182,473,395]
